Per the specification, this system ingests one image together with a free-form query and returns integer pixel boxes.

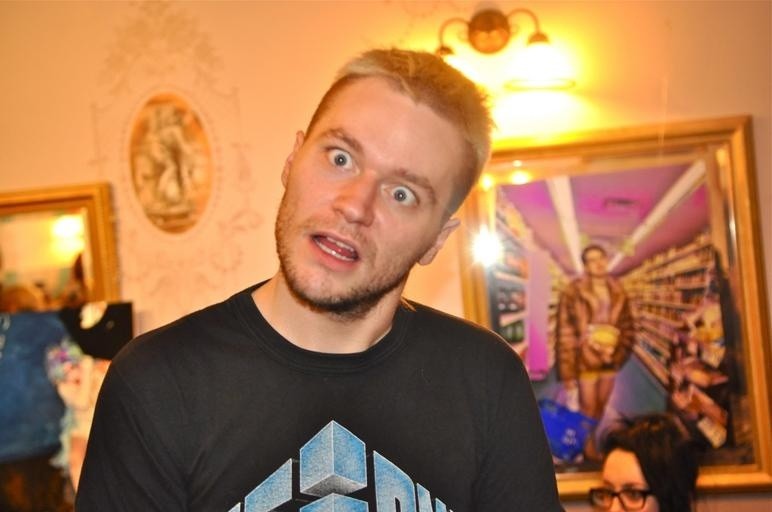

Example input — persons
[68,50,580,512]
[554,246,636,466]
[589,410,711,512]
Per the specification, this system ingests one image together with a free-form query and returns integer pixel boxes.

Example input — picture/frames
[1,183,120,314]
[455,116,771,500]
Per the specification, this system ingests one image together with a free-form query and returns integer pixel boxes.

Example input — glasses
[588,487,652,511]
